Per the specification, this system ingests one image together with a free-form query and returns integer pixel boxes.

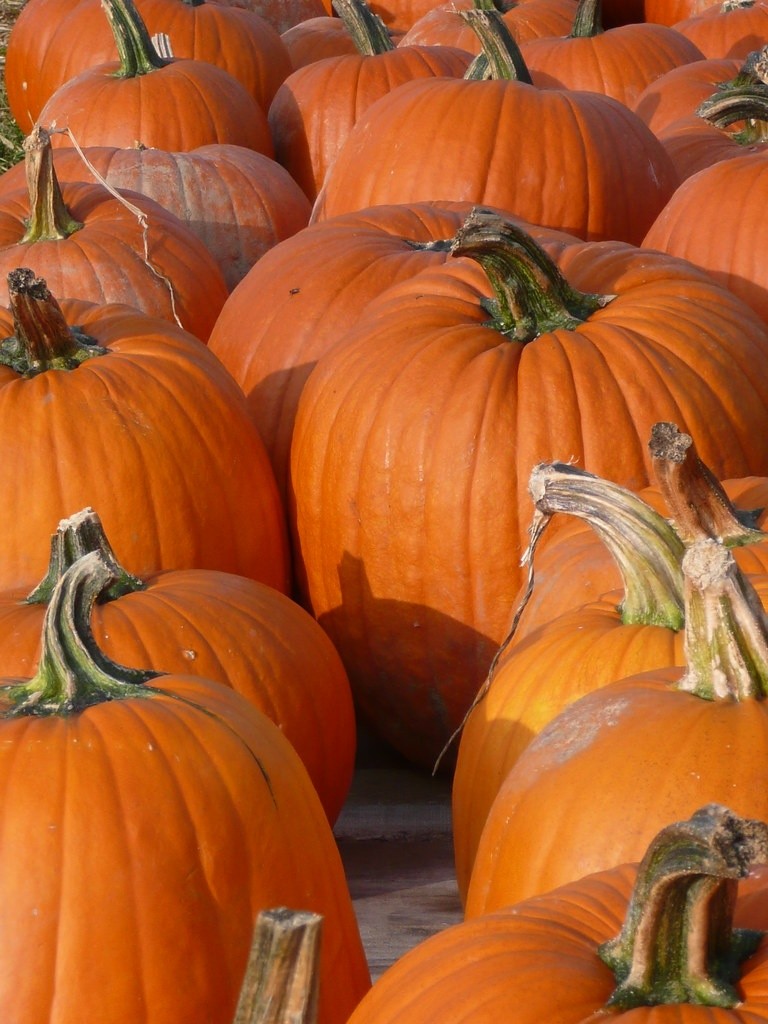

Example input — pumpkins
[0,1,768,1024]
